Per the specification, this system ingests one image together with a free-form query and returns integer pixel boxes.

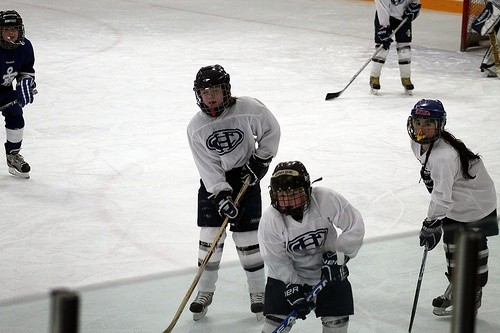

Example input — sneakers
[249,292,265,321]
[189,291,214,322]
[432,283,454,317]
[3,140,31,179]
[369,76,381,95]
[401,78,414,96]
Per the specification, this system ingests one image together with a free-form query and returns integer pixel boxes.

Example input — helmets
[193,64,232,117]
[406,98,447,144]
[0,10,26,49]
[269,161,312,215]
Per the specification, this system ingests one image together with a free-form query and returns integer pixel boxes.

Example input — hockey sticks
[0,89,38,111]
[407,241,429,333]
[271,278,328,333]
[325,16,409,100]
[483,0,500,71]
[163,174,251,333]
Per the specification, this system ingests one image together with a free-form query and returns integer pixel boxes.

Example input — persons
[407,99,499,317]
[258,160,365,333]
[369,0,422,90]
[0,9,36,173]
[469,0,500,78]
[187,64,281,314]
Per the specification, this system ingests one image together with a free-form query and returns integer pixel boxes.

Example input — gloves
[207,188,242,227]
[376,26,394,50]
[239,155,273,187]
[284,284,315,321]
[402,3,421,22]
[15,75,36,107]
[320,251,350,286]
[419,220,443,251]
